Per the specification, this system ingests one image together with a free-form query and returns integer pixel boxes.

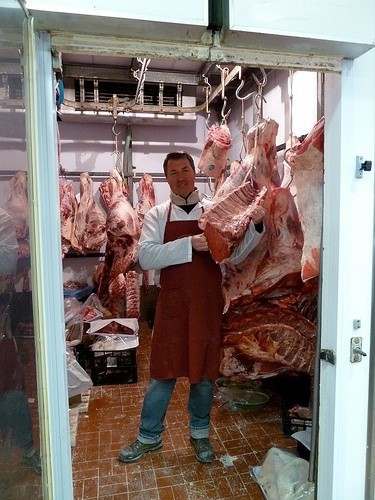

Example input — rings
[255,211,258,214]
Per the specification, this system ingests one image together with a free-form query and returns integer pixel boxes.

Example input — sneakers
[189,432,215,464]
[118,437,158,465]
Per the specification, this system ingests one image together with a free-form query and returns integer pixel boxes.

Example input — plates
[230,390,270,410]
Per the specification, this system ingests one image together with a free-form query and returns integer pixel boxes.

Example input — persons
[113,148,266,464]
[0,207,42,474]
